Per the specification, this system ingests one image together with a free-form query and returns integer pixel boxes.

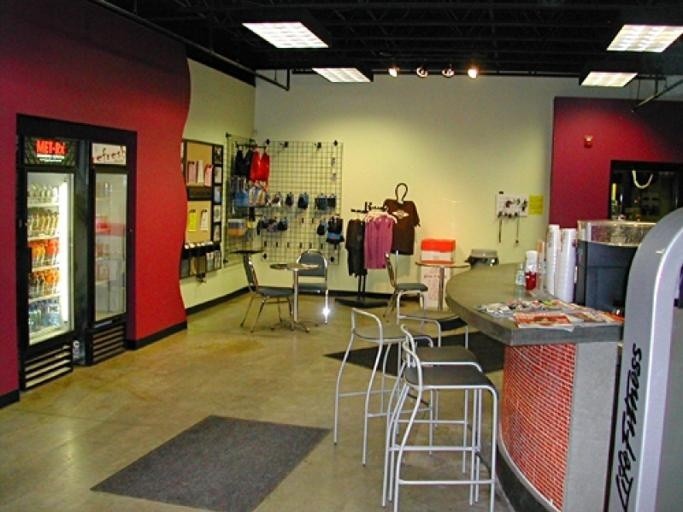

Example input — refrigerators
[13,115,136,394]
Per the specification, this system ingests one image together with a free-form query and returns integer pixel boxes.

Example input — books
[473,299,624,333]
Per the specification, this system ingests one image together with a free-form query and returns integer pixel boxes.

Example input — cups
[515,223,579,303]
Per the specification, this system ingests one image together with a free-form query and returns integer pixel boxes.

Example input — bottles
[27,207,58,238]
[27,240,59,267]
[27,300,59,332]
[95,178,112,281]
[26,184,59,205]
[29,268,58,299]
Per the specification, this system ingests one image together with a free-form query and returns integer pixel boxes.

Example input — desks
[268,262,319,335]
[414,260,470,321]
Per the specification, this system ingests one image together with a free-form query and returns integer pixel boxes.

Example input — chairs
[332,308,441,470]
[237,257,296,333]
[381,322,487,508]
[292,250,331,326]
[378,340,500,511]
[381,250,433,317]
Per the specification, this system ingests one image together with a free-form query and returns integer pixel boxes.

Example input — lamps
[309,53,373,86]
[387,55,482,80]
[597,25,682,56]
[240,21,329,50]
[578,61,638,88]
